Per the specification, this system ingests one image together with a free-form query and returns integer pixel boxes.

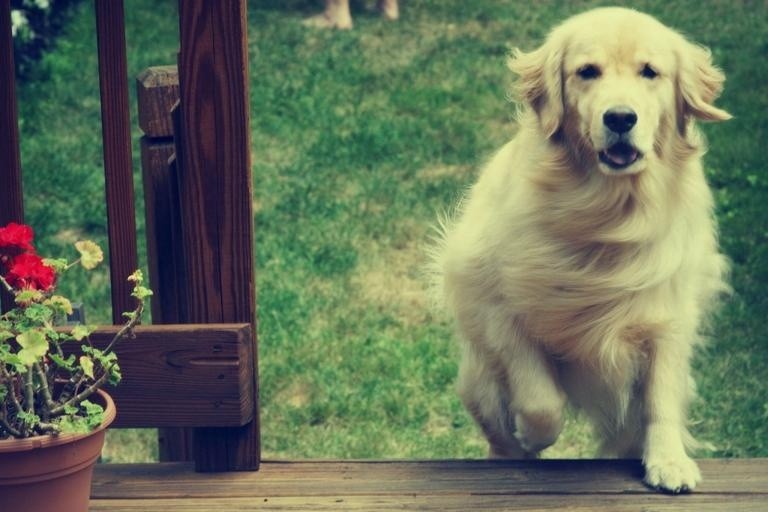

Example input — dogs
[417,4,735,496]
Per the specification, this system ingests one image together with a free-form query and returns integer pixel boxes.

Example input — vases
[0,389,118,512]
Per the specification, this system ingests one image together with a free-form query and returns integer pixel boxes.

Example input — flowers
[0,222,153,439]
